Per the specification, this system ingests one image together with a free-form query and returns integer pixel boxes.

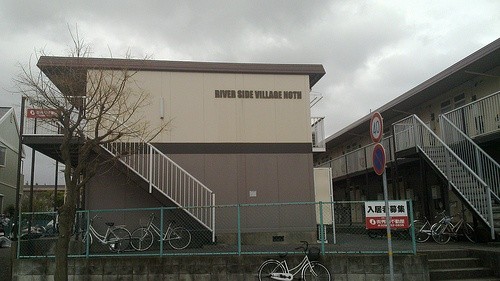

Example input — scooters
[362,197,407,240]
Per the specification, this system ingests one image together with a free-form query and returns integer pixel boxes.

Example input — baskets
[308,247,321,260]
[79,218,93,231]
[139,215,151,227]
[456,212,467,221]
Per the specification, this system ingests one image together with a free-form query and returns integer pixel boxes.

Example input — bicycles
[430,208,479,244]
[407,210,459,243]
[129,211,192,250]
[74,209,132,253]
[257,240,332,281]
